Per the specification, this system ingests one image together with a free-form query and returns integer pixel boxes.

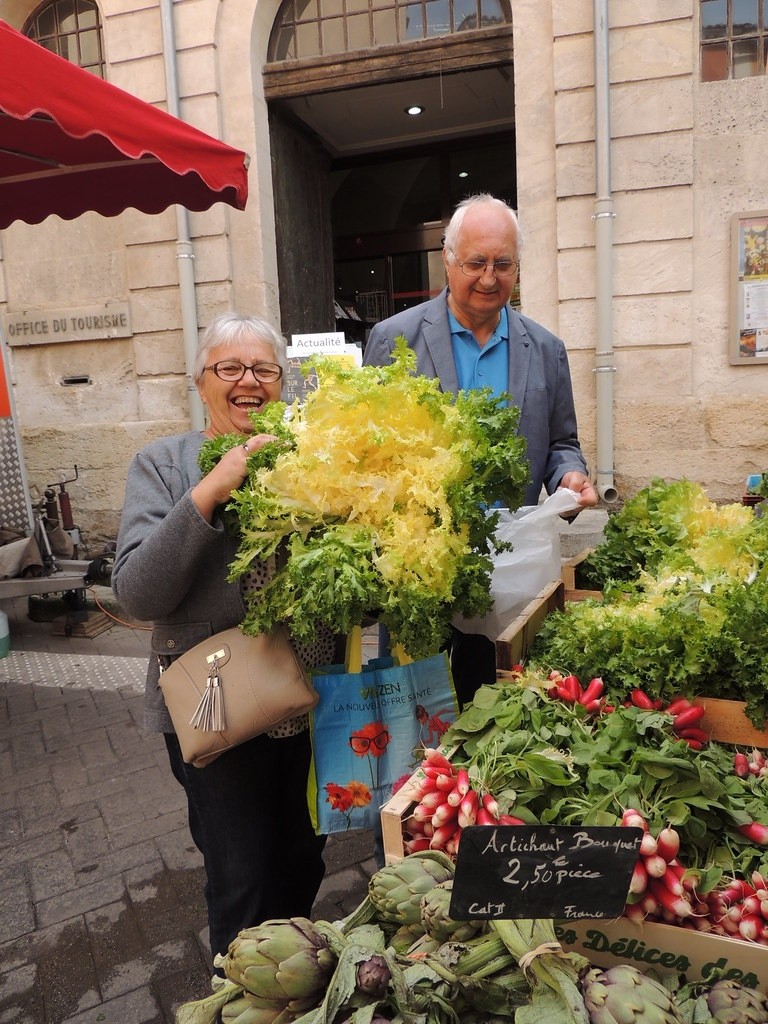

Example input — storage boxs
[375,543,768,1003]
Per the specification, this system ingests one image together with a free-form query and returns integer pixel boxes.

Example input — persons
[361,193,600,711]
[112,311,334,979]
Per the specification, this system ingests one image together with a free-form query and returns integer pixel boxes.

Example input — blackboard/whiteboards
[448,824,646,919]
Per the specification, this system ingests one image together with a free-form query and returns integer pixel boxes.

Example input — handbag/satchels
[157,623,320,770]
[306,625,461,837]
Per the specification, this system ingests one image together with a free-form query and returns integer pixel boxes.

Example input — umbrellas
[0,23,262,268]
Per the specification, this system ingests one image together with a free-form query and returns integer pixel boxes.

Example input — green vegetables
[194,333,530,658]
[523,476,768,736]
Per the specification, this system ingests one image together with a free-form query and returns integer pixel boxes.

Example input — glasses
[204,360,283,384]
[449,247,519,277]
